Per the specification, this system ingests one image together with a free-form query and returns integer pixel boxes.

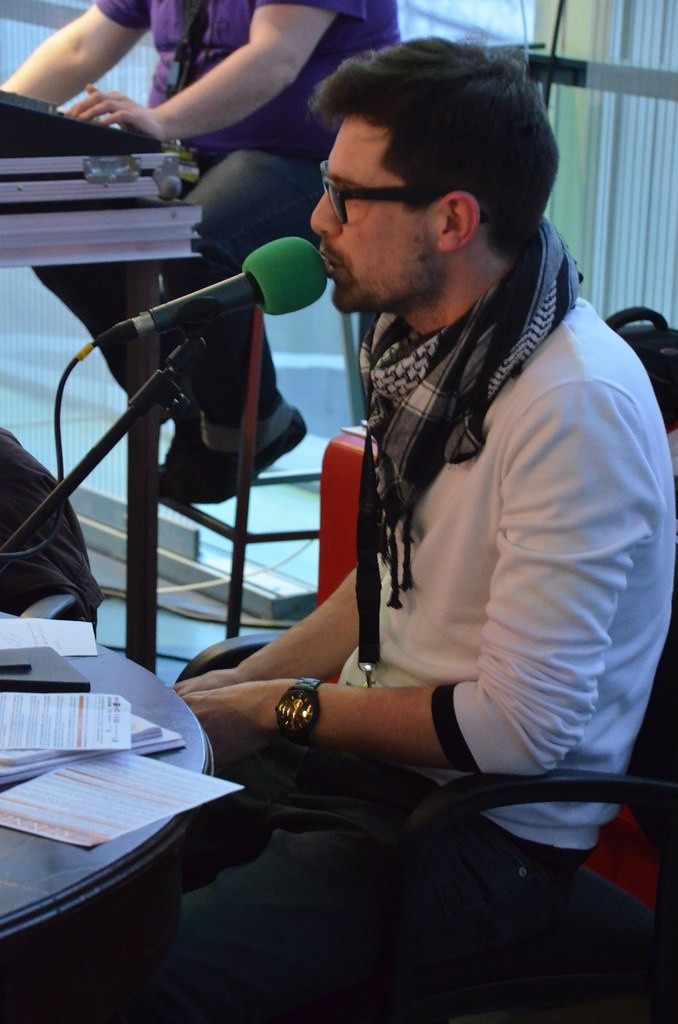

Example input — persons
[109,38,675,1024]
[0,0,402,502]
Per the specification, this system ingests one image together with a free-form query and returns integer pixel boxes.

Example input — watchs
[275,677,324,748]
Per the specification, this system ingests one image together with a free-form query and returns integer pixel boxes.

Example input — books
[0,690,186,786]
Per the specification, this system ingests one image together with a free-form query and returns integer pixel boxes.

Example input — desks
[0,611,216,1024]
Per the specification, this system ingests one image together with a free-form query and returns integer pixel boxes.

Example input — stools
[158,306,366,639]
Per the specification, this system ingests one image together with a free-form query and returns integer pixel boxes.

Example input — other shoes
[157,404,307,503]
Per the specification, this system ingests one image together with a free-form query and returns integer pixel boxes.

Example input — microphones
[96,236,328,347]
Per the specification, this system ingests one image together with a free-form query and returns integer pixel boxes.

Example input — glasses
[319,160,487,224]
[172,0,209,69]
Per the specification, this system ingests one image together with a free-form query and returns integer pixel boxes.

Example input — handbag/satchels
[605,306,678,425]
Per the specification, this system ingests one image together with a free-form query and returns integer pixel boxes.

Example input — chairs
[173,564,678,1024]
[0,427,105,638]
